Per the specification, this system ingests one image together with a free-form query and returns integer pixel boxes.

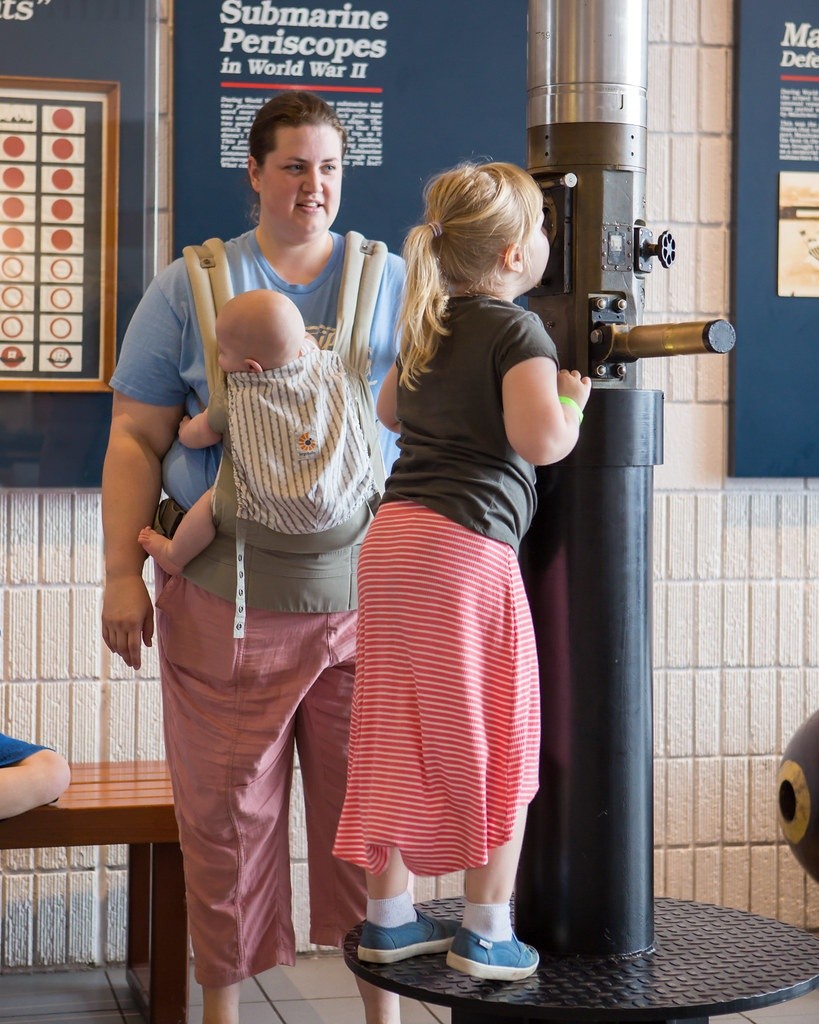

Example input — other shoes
[357,907,463,963]
[445,926,540,981]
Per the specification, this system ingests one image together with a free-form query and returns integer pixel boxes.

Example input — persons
[139,291,375,576]
[102,92,407,1024]
[333,162,591,979]
[1,733,71,819]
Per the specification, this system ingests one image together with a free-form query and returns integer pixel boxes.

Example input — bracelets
[561,397,584,420]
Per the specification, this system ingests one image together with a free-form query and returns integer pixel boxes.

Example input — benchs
[0,758,189,1024]
